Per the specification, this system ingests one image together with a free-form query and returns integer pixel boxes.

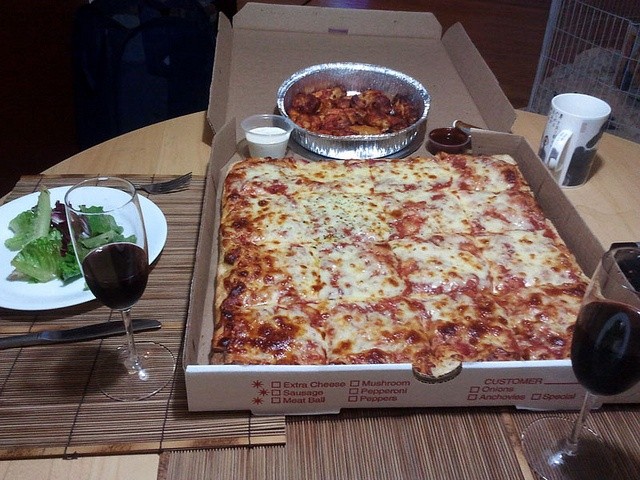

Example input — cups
[428,127,469,153]
[535,92,612,188]
[241,113,295,160]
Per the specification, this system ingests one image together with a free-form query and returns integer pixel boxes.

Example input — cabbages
[4,185,64,283]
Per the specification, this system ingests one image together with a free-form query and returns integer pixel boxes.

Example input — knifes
[0,320,162,349]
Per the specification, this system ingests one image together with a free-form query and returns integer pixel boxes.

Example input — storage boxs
[527,1,640,145]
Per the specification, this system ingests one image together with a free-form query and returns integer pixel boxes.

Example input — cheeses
[246,126,290,161]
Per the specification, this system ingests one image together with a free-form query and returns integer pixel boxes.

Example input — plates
[0,183,167,310]
[276,61,431,160]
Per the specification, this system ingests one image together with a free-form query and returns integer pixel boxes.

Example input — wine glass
[520,249,640,476]
[64,178,177,403]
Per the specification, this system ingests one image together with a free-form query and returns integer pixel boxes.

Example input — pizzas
[208,152,606,364]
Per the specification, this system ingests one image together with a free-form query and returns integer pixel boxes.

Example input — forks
[57,171,191,192]
